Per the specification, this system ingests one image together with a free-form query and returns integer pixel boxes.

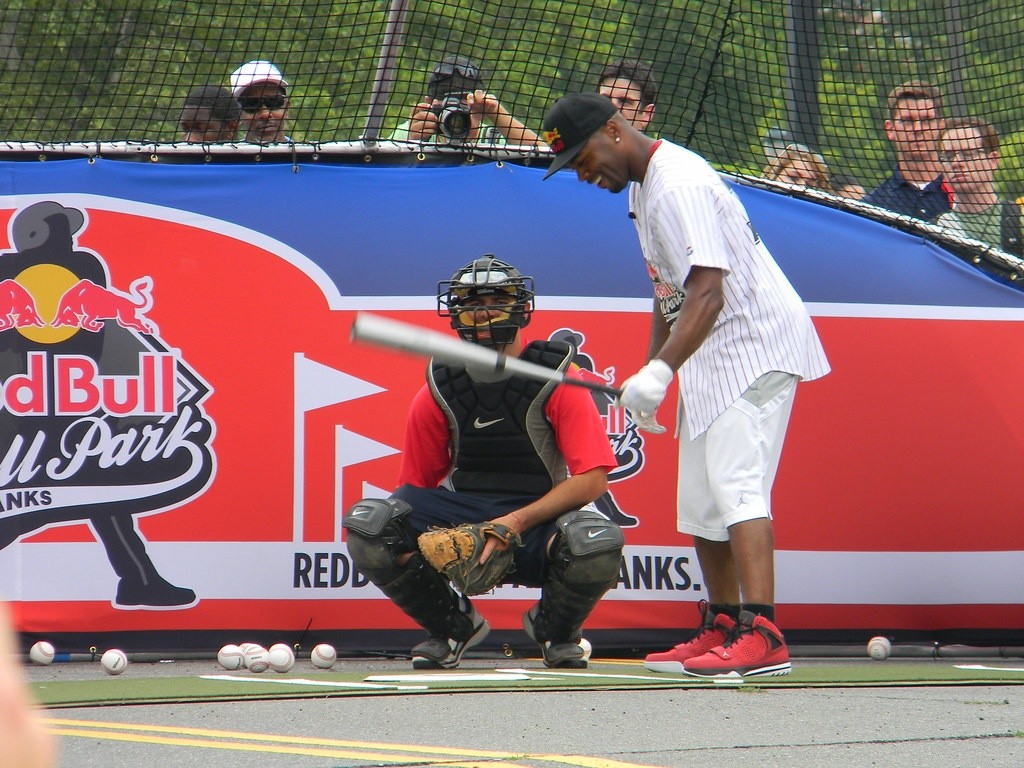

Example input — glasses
[238,95,286,112]
[428,63,482,91]
[776,144,827,173]
[943,148,986,161]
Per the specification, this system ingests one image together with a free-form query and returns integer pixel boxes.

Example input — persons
[830,175,865,199]
[599,60,657,133]
[864,78,957,223]
[340,253,624,670]
[180,86,238,142]
[761,144,830,190]
[543,96,832,679]
[388,54,547,146]
[231,61,292,142]
[931,119,1024,259]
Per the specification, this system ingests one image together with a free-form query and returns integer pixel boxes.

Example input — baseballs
[577,637,591,662]
[866,636,891,660]
[310,643,337,670]
[30,641,54,666]
[217,643,296,674]
[100,649,128,676]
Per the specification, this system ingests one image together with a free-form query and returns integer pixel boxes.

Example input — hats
[427,57,484,99]
[230,60,289,101]
[543,93,618,179]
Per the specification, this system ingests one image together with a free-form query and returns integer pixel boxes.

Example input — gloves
[613,359,673,417]
[632,410,667,434]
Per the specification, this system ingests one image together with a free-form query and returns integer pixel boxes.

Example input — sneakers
[523,602,588,668]
[411,596,490,669]
[644,599,738,674]
[682,610,792,677]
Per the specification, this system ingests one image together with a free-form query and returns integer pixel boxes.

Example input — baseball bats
[348,309,627,399]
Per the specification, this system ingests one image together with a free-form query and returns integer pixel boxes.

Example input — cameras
[425,98,473,138]
